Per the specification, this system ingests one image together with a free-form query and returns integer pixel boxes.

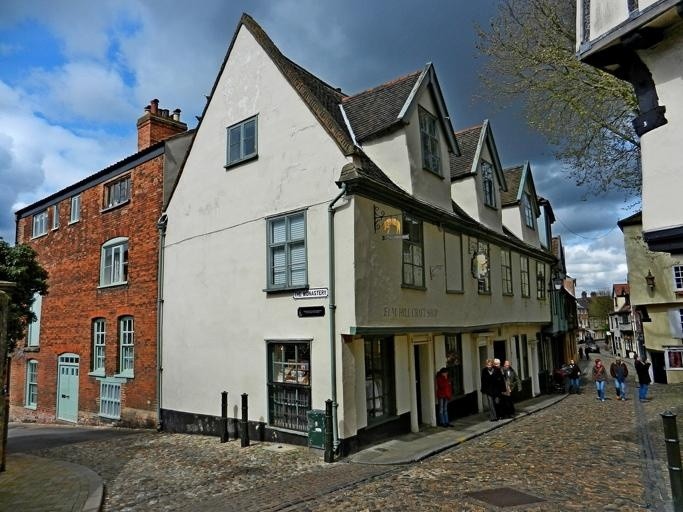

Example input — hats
[616,357,621,360]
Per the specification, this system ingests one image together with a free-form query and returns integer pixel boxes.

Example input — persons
[578,345,583,359]
[566,359,582,395]
[610,356,629,401]
[591,359,609,401]
[584,345,589,360]
[480,357,523,419]
[435,367,455,428]
[632,354,652,402]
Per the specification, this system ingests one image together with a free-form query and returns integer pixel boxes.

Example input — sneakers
[598,397,605,402]
[439,423,454,428]
[640,399,649,401]
[616,395,625,401]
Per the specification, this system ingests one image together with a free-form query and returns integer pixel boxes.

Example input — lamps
[549,276,562,291]
[645,269,656,288]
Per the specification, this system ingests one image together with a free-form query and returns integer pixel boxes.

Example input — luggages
[500,392,515,415]
